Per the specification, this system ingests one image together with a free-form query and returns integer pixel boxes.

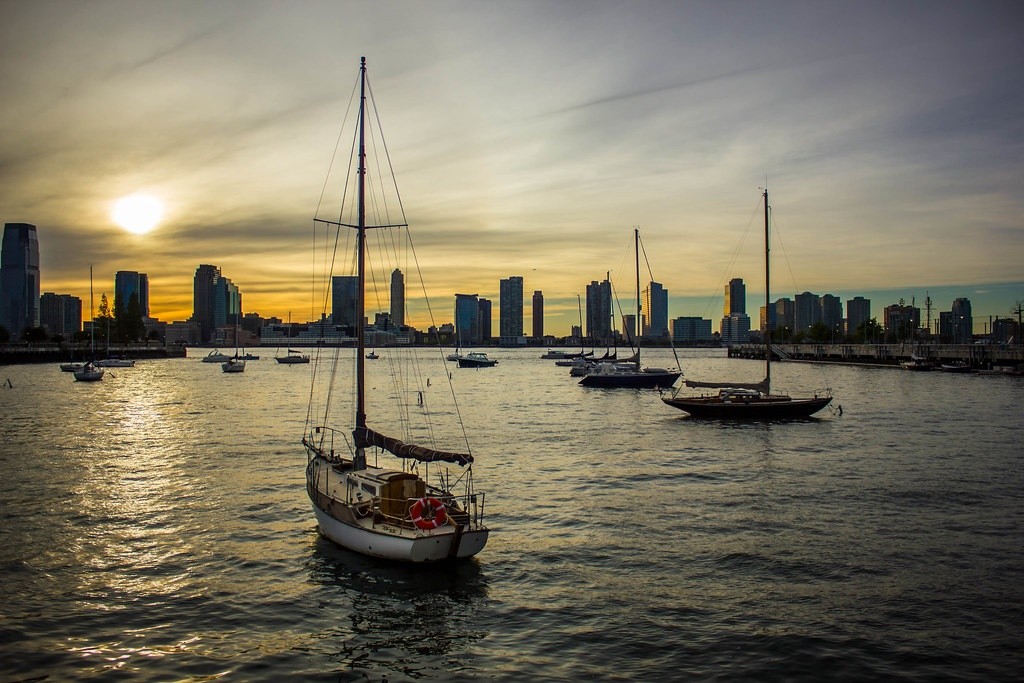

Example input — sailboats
[59,264,136,380]
[301,56,491,564]
[457,306,499,368]
[659,186,835,421]
[541,227,684,390]
[273,311,310,363]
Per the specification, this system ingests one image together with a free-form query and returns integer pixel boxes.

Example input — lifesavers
[411,497,448,530]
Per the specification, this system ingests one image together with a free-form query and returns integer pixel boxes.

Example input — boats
[365,350,380,360]
[447,347,464,362]
[201,304,260,372]
